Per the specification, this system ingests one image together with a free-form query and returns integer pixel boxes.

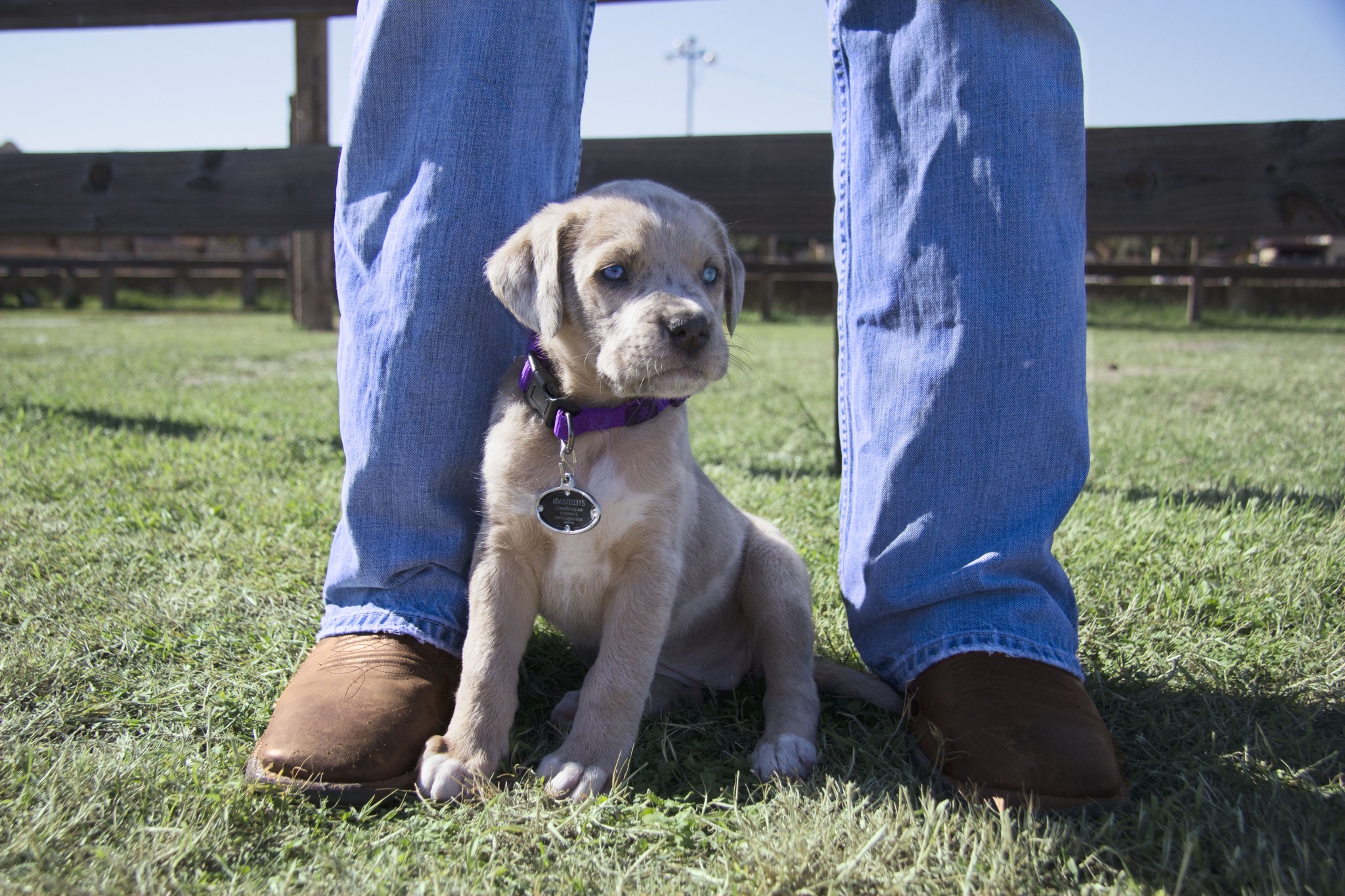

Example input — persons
[242,1,1132,812]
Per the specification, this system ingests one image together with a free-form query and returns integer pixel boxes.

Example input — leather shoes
[910,651,1125,811]
[247,633,458,804]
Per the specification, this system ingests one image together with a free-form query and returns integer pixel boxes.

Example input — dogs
[414,180,903,811]
